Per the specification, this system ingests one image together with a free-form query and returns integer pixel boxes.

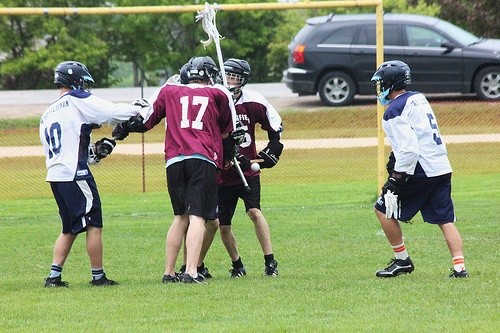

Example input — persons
[370,60,470,279]
[149,63,246,279]
[40,61,150,288]
[198,58,284,279]
[113,56,236,282]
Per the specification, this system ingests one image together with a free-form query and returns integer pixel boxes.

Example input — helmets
[54,60,94,90]
[370,60,411,105]
[219,58,252,80]
[180,56,217,85]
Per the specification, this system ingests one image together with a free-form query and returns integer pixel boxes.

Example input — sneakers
[44,277,69,288]
[163,272,182,283]
[264,259,278,276]
[376,255,414,277]
[449,268,469,278]
[202,267,212,278]
[179,264,186,275]
[91,274,119,285]
[182,272,205,283]
[231,268,246,277]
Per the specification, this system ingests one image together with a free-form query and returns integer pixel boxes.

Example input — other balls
[251,163,260,172]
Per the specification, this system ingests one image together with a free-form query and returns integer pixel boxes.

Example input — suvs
[281,12,500,106]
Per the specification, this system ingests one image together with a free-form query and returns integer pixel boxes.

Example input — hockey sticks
[90,135,122,165]
[161,74,254,192]
[200,1,238,137]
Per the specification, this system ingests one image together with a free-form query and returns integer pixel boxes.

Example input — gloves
[222,136,235,160]
[112,122,129,140]
[257,139,283,169]
[131,98,149,107]
[384,177,401,220]
[129,115,144,132]
[95,137,116,158]
[233,152,250,172]
